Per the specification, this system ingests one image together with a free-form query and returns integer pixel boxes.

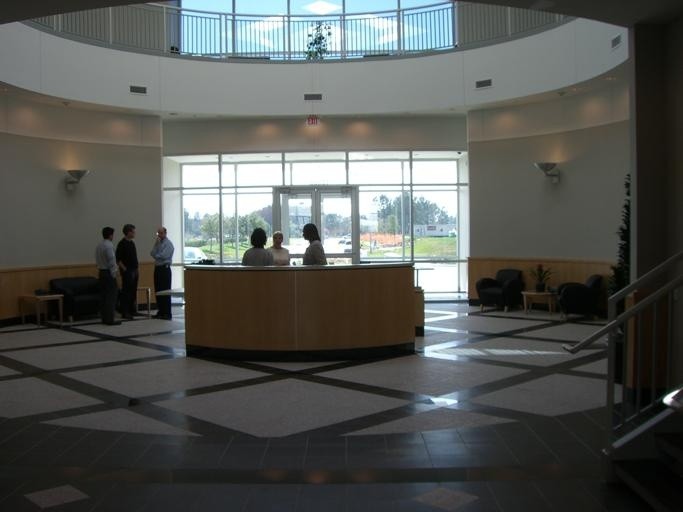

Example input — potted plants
[606,172,631,385]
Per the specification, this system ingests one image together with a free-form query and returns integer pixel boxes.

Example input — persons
[115,223,147,322]
[241,227,274,265]
[95,227,123,325]
[302,223,327,265]
[269,230,290,265]
[150,227,175,321]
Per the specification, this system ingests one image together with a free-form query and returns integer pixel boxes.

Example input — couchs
[50,277,121,323]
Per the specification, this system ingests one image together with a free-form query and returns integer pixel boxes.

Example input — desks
[18,294,66,328]
[521,289,559,315]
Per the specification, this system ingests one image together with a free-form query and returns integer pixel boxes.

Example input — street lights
[296,202,304,225]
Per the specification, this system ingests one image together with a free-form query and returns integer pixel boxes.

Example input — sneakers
[103,310,172,325]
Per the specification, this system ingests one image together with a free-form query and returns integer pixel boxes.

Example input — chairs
[558,275,604,321]
[476,269,523,313]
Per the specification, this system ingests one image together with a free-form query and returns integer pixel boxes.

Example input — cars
[339,234,352,253]
[185,246,206,260]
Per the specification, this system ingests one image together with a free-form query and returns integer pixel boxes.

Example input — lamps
[65,170,90,192]
[534,161,562,185]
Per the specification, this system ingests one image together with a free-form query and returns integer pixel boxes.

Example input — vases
[536,283,545,292]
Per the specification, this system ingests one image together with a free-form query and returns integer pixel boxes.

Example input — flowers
[528,264,552,284]
